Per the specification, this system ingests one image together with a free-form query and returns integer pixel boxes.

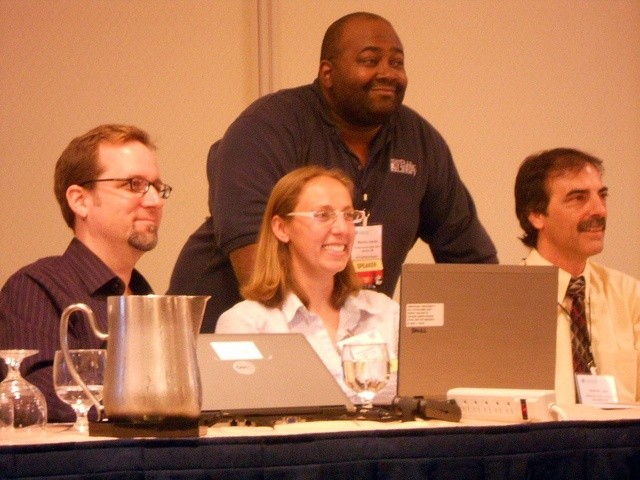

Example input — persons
[213,164,400,405]
[0,124,173,423]
[514,147,640,404]
[164,11,500,333]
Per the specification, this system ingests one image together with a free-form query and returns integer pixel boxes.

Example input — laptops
[398,260,558,409]
[189,334,358,415]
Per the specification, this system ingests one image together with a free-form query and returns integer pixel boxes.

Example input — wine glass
[342,343,392,422]
[54,350,106,439]
[0,349,48,433]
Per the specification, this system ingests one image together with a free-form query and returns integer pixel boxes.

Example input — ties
[567,275,596,403]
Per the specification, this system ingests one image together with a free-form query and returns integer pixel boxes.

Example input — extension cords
[444,387,558,423]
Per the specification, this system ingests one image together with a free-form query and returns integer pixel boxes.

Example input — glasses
[286,209,366,224]
[77,176,173,198]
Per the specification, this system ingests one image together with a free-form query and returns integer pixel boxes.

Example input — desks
[0,408,640,480]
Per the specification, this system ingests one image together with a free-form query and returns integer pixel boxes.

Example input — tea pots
[60,294,211,436]
